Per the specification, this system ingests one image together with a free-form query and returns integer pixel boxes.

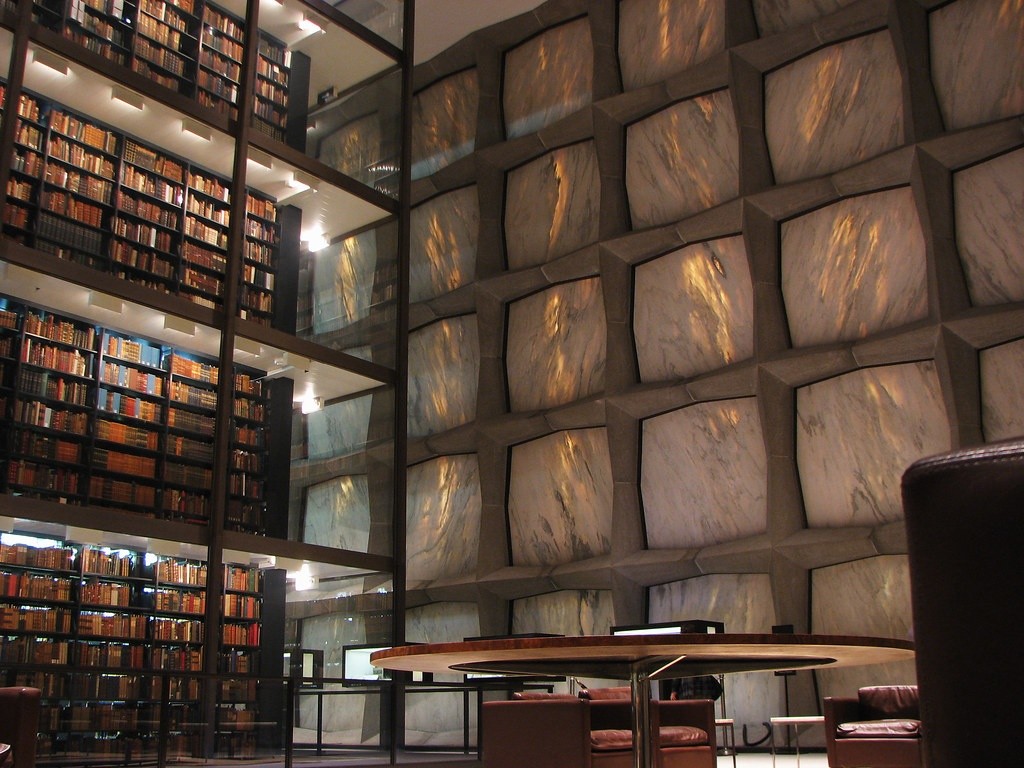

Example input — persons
[670,675,722,702]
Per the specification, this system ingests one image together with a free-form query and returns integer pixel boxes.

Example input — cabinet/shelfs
[0,0,413,758]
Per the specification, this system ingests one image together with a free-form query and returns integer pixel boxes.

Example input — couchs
[479,687,717,768]
[0,686,41,768]
[819,684,921,768]
[899,436,1024,768]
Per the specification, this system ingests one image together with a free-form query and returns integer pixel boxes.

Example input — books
[0,533,263,755]
[0,82,276,326]
[0,303,268,534]
[1,1,288,148]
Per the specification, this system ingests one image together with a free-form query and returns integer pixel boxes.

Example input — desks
[770,716,824,758]
[714,716,736,768]
[369,633,915,768]
